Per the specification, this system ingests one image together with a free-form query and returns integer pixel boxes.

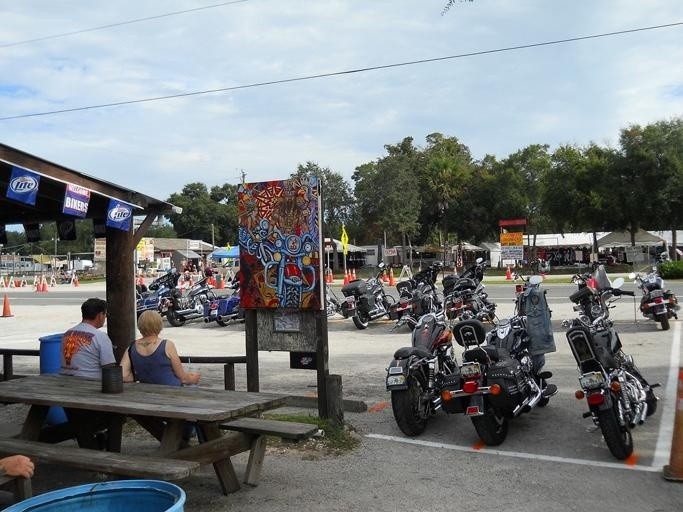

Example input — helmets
[453,278,476,291]
[432,261,444,269]
[205,267,214,276]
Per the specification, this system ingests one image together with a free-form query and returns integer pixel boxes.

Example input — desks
[0,372,291,496]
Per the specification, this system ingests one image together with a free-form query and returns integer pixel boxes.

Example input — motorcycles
[134,267,218,327]
[390,261,442,331]
[562,287,661,459]
[339,258,392,330]
[440,257,495,323]
[204,270,245,326]
[569,247,616,320]
[628,251,680,331]
[385,289,557,446]
[514,274,531,317]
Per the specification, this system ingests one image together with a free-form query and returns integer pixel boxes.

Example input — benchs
[146,416,317,489]
[1,433,205,491]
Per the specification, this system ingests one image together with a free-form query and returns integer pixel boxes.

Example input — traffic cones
[15,279,18,287]
[36,280,48,292]
[389,268,396,286]
[4,276,9,287]
[344,268,356,286]
[0,294,14,317]
[75,281,79,287]
[383,269,388,282]
[219,272,224,289]
[505,266,513,280]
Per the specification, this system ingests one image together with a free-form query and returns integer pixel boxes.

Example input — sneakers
[95,429,108,443]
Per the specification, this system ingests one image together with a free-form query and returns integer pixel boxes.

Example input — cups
[189,368,201,387]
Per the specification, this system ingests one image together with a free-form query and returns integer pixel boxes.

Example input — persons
[0,454,34,480]
[135,257,240,289]
[58,298,116,379]
[119,311,206,444]
